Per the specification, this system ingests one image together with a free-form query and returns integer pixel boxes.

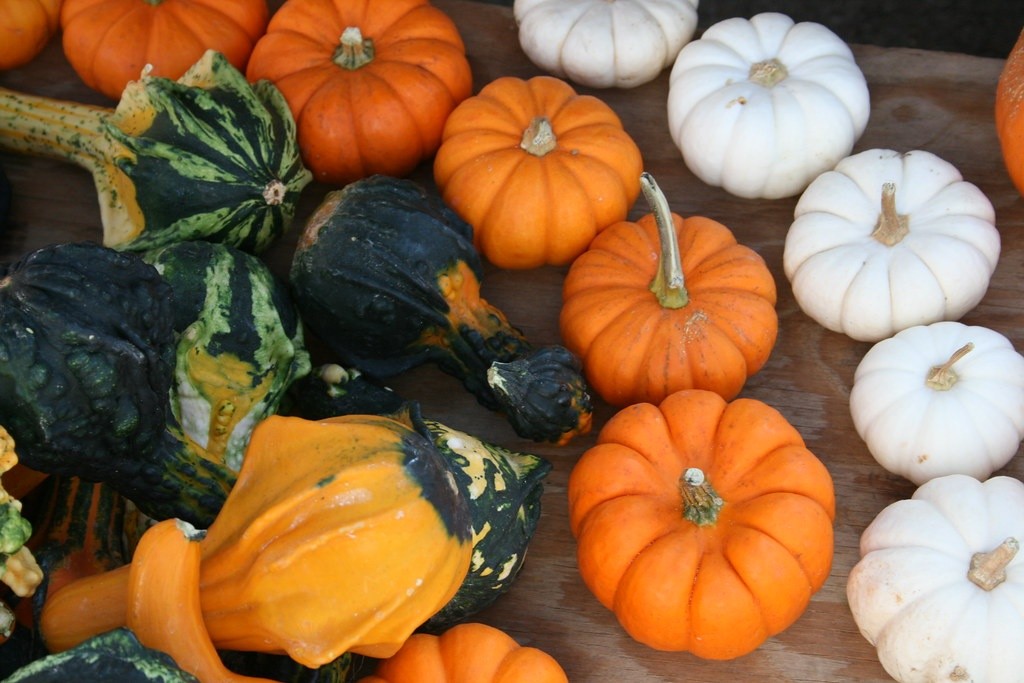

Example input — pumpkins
[0,0,1024,683]
